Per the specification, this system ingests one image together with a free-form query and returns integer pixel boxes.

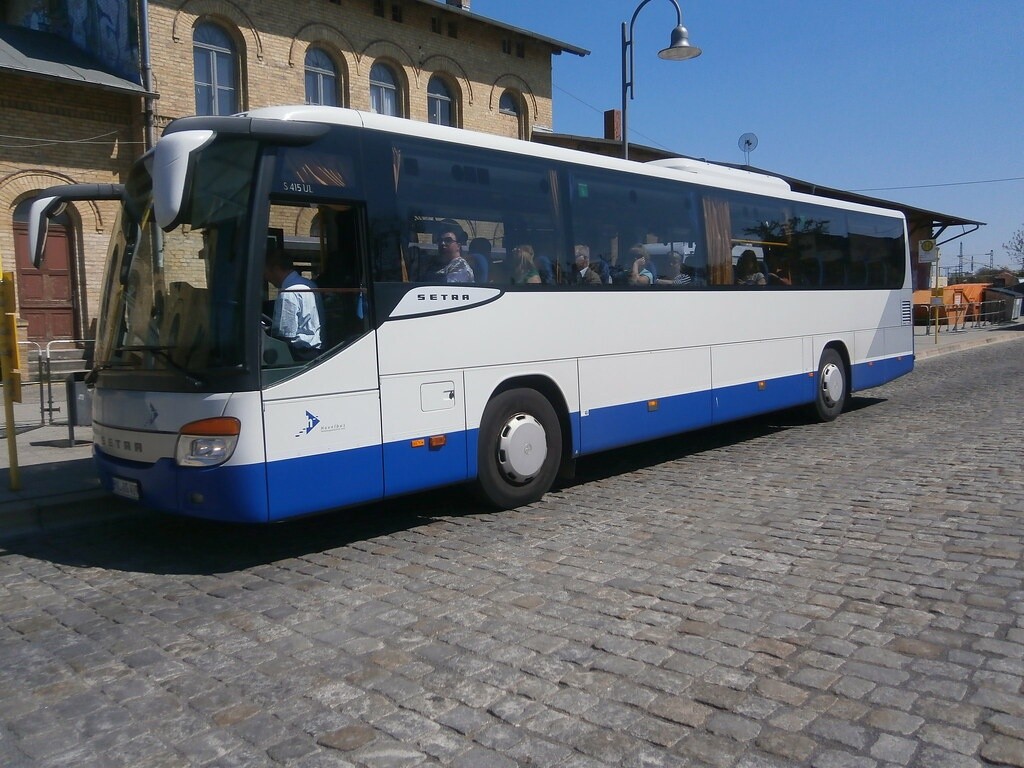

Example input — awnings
[0,38,160,113]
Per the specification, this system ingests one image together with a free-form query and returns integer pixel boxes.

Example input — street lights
[622,0,703,161]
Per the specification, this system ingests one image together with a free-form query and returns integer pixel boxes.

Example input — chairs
[804,257,824,288]
[825,262,848,289]
[848,255,869,289]
[759,261,769,279]
[646,260,657,283]
[590,258,611,284]
[865,256,888,289]
[534,254,554,283]
[471,251,489,283]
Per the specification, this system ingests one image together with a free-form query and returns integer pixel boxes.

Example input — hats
[431,219,468,247]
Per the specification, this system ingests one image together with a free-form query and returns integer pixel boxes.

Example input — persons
[591,217,638,278]
[265,251,328,354]
[767,263,792,285]
[420,231,475,284]
[656,252,692,285]
[613,245,654,286]
[509,243,542,284]
[736,250,766,285]
[573,244,602,286]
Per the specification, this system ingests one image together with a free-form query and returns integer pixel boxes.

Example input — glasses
[628,253,643,260]
[436,237,460,244]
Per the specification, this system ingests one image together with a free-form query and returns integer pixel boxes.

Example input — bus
[29,105,915,525]
